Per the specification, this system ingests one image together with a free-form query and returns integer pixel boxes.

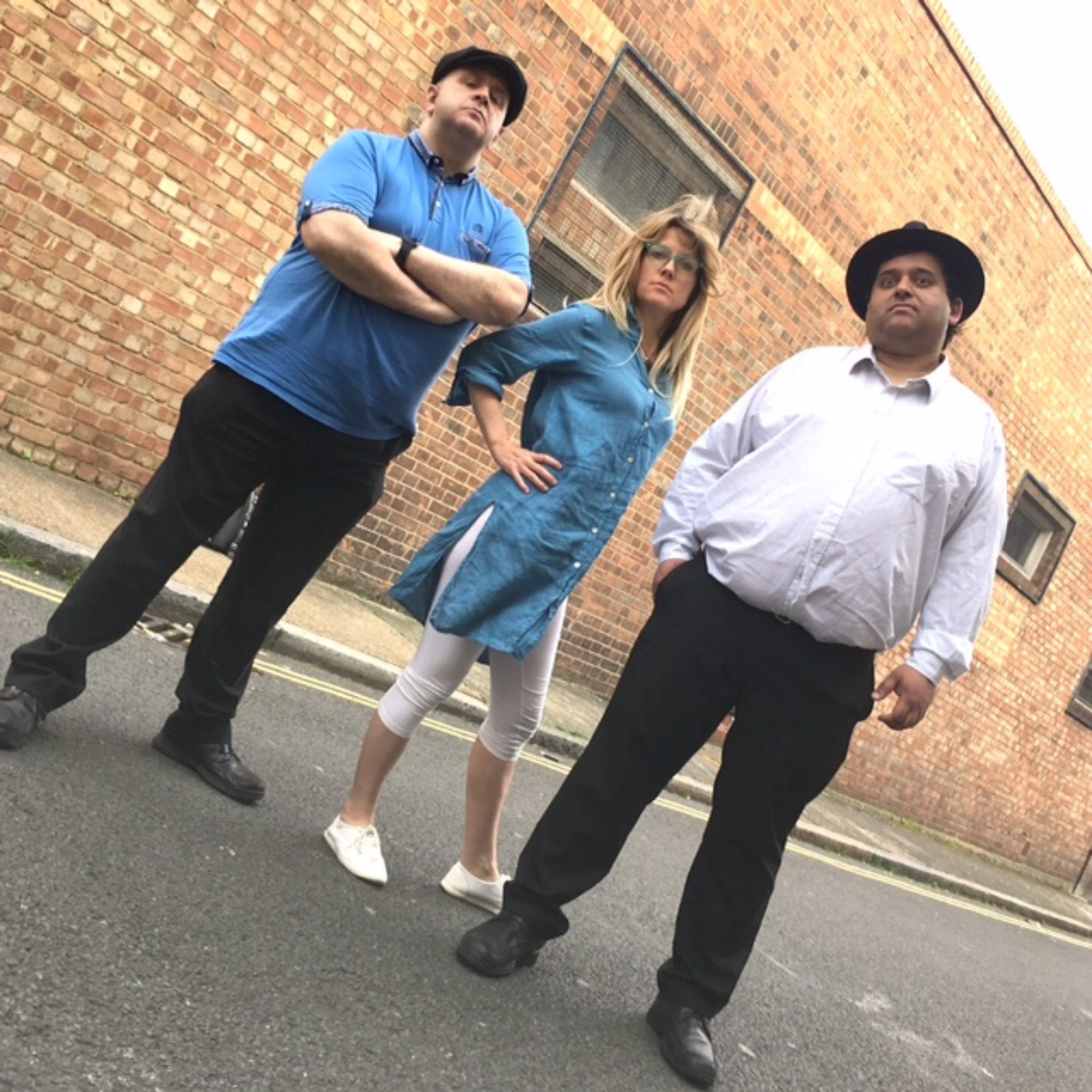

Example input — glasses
[645,243,704,275]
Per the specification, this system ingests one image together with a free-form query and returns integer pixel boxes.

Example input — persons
[0,45,535,806]
[320,193,728,918]
[454,221,1011,1086]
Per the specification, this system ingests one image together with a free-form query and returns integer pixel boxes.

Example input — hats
[430,45,528,127]
[845,221,986,325]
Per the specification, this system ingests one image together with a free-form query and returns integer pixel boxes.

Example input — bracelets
[393,236,423,271]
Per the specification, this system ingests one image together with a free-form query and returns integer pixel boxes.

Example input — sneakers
[440,860,514,915]
[322,814,387,885]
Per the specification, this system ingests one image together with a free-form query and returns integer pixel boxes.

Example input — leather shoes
[151,730,266,803]
[645,990,718,1086]
[455,907,548,976]
[0,683,47,749]
